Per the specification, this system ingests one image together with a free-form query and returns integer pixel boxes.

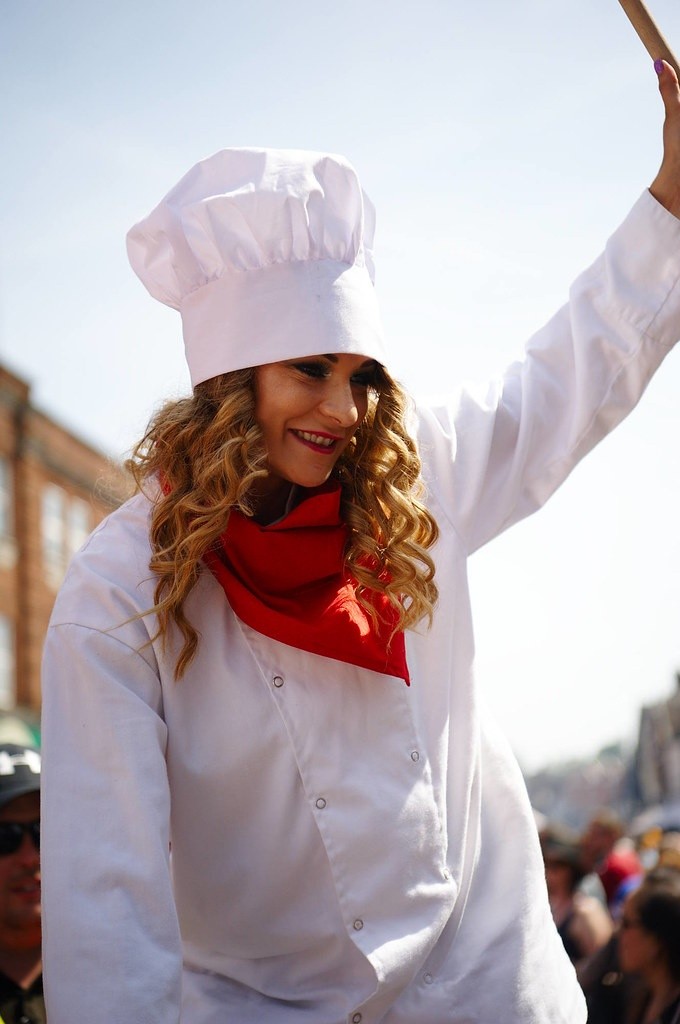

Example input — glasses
[0,819,40,856]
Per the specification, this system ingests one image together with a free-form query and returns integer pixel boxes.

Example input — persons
[0,743,48,1023]
[534,804,680,1024]
[39,60,680,1024]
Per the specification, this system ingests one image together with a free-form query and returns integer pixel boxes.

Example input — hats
[0,744,41,803]
[127,148,392,386]
